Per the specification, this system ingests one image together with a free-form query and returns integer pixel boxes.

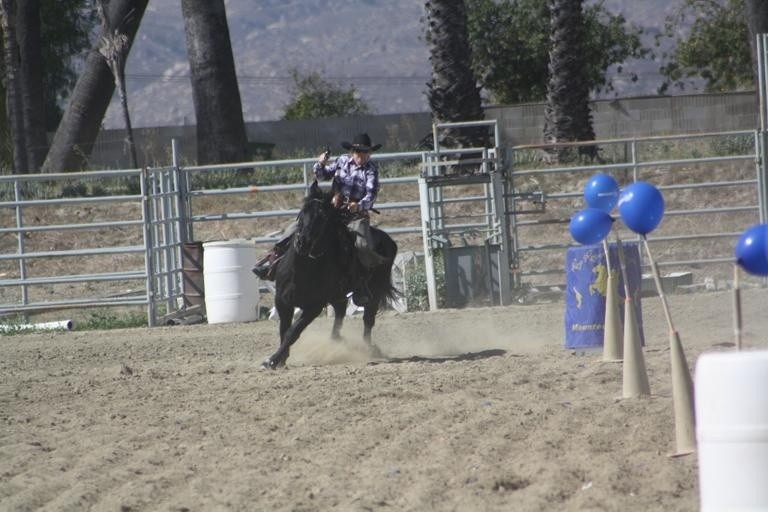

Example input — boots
[251,246,286,281]
[354,263,373,304]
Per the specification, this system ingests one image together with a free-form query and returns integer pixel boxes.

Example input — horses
[260,178,405,376]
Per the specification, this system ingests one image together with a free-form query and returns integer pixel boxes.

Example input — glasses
[353,148,369,154]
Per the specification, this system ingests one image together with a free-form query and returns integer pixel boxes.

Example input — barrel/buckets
[564,241,645,349]
[202,238,260,324]
[695,349,768,512]
[181,241,205,316]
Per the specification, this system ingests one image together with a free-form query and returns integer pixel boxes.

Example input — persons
[251,133,382,307]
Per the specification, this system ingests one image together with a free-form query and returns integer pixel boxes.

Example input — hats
[341,133,381,151]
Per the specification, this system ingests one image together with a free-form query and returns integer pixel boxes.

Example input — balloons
[582,172,621,213]
[569,206,616,247]
[615,178,664,239]
[735,222,767,278]
